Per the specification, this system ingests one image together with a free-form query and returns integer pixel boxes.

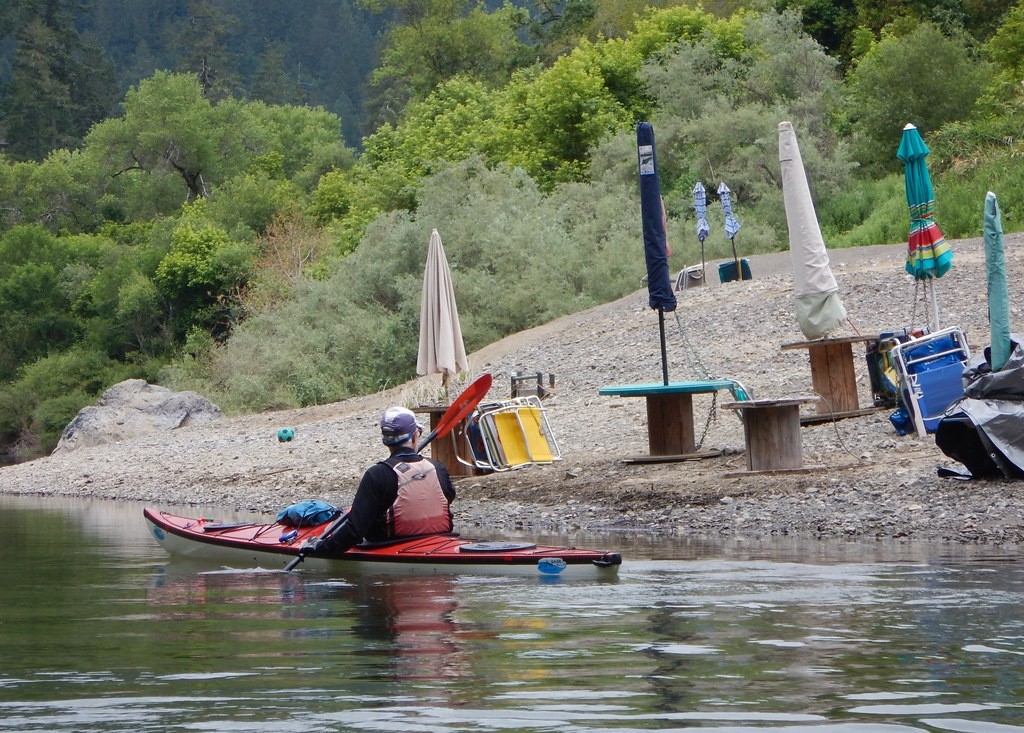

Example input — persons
[300,406,456,554]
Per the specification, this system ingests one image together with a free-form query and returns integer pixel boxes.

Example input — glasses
[408,426,422,439]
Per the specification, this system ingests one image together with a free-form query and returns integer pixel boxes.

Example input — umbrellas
[985,191,1012,372]
[693,180,711,283]
[636,121,677,385]
[416,228,469,406]
[896,123,954,333]
[777,120,847,340]
[717,180,742,281]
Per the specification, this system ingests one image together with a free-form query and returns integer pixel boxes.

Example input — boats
[931,189,1024,482]
[141,506,624,580]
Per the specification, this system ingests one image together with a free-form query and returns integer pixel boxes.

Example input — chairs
[676,264,704,291]
[891,327,972,439]
[451,396,562,474]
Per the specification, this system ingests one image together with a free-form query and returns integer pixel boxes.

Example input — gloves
[299,535,322,557]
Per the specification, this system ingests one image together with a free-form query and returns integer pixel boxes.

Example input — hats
[382,406,417,446]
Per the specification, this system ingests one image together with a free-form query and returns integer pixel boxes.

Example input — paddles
[282,374,493,571]
